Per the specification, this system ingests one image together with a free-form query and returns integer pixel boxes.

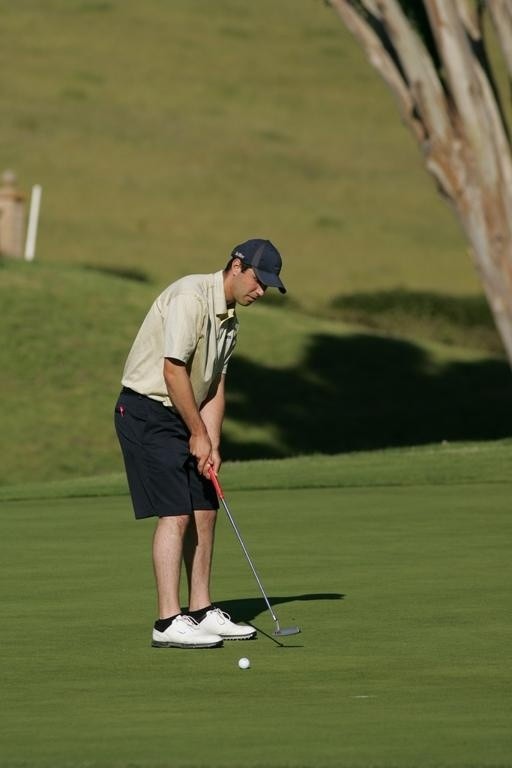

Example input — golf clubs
[207,467,300,636]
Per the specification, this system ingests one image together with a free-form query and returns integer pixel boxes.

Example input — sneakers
[149,611,222,650]
[191,608,256,642]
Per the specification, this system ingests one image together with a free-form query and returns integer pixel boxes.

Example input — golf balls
[239,658,249,669]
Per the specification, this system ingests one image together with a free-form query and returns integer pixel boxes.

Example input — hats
[230,239,286,295]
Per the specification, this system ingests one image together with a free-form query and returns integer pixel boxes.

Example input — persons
[112,237,286,651]
